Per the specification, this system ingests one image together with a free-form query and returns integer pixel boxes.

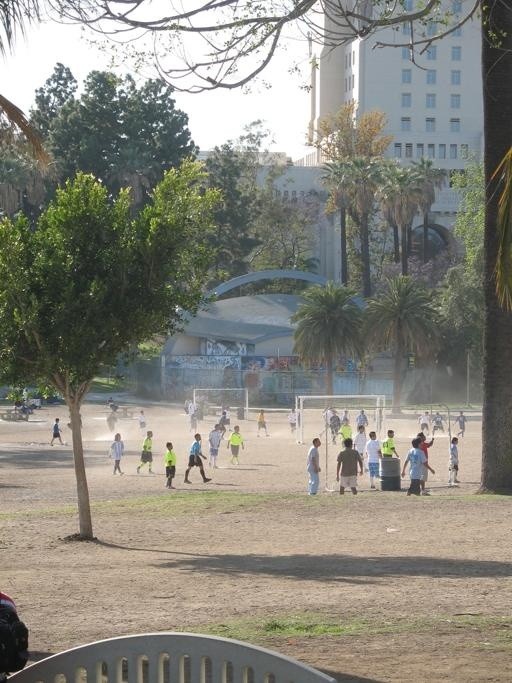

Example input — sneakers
[421,492,431,496]
[136,467,155,474]
[203,478,211,483]
[183,479,192,483]
[163,483,175,489]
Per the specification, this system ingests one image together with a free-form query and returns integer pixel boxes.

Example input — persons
[306,438,321,495]
[184,433,212,484]
[164,442,176,489]
[288,409,304,434]
[109,433,124,476]
[139,410,145,433]
[208,424,221,469]
[217,405,231,440]
[319,408,435,497]
[257,409,270,437]
[184,395,204,433]
[136,431,155,474]
[448,438,460,483]
[227,426,244,465]
[452,411,467,438]
[50,418,65,447]
[15,399,21,407]
[432,413,446,436]
[107,414,117,434]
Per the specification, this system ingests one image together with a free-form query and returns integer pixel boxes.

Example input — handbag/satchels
[0,606,28,672]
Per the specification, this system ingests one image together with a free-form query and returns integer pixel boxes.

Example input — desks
[118,405,133,417]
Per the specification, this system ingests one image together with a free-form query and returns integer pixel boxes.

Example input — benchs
[0,409,30,422]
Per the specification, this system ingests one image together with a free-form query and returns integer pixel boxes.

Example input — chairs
[7,632,337,683]
